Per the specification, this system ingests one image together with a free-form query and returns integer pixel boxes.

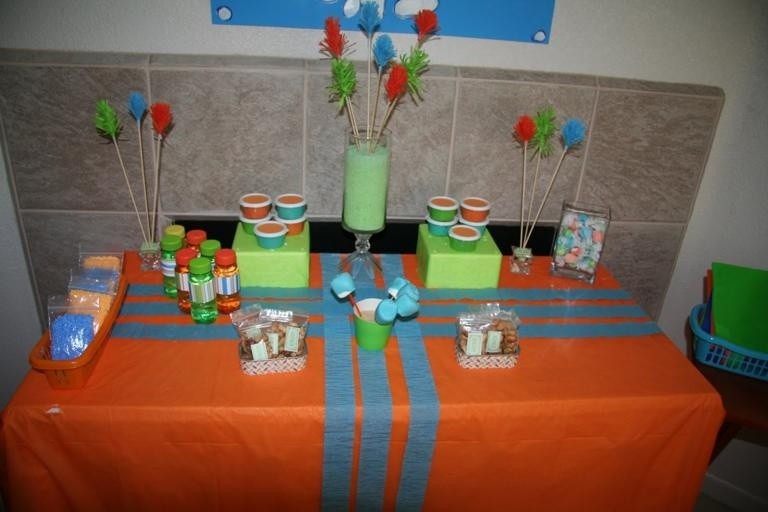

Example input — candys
[554,213,605,274]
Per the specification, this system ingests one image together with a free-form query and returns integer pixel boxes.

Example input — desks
[1,253,726,512]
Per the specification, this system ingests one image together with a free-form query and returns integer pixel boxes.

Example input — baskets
[689,304,768,381]
[30,275,128,388]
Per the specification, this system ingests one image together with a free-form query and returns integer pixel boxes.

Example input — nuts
[459,319,517,354]
[239,322,307,359]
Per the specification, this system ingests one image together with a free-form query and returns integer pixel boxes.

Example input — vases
[338,125,392,277]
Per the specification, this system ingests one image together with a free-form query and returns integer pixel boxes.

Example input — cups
[351,297,395,353]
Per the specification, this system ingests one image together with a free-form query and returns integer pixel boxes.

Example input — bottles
[185,229,208,259]
[212,249,240,314]
[200,239,221,272]
[187,256,217,324]
[160,235,183,298]
[175,249,198,314]
[163,225,186,248]
